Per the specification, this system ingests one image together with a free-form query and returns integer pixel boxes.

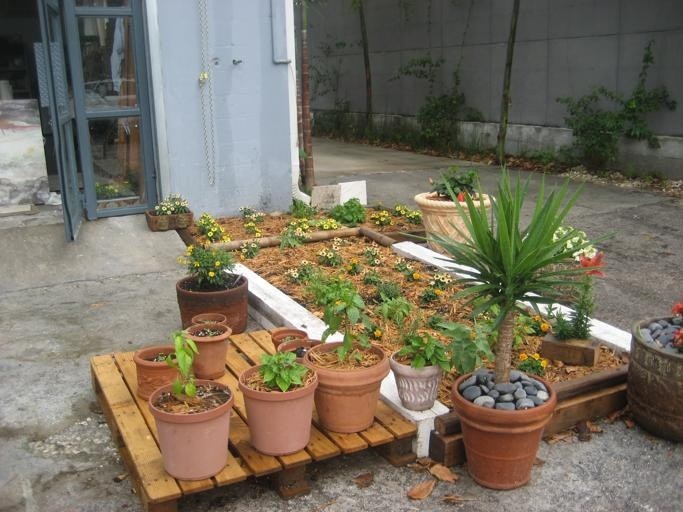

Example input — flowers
[429,166,483,202]
[174,240,236,289]
[668,300,682,353]
[150,191,190,216]
[521,223,601,261]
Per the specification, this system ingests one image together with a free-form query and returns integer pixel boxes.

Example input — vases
[175,273,250,335]
[529,256,586,305]
[627,315,682,443]
[144,208,193,232]
[414,192,495,260]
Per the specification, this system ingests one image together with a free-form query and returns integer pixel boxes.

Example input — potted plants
[388,331,451,413]
[184,309,233,381]
[268,326,324,364]
[431,163,621,492]
[150,330,238,482]
[234,350,320,458]
[303,271,393,436]
[134,343,183,402]
